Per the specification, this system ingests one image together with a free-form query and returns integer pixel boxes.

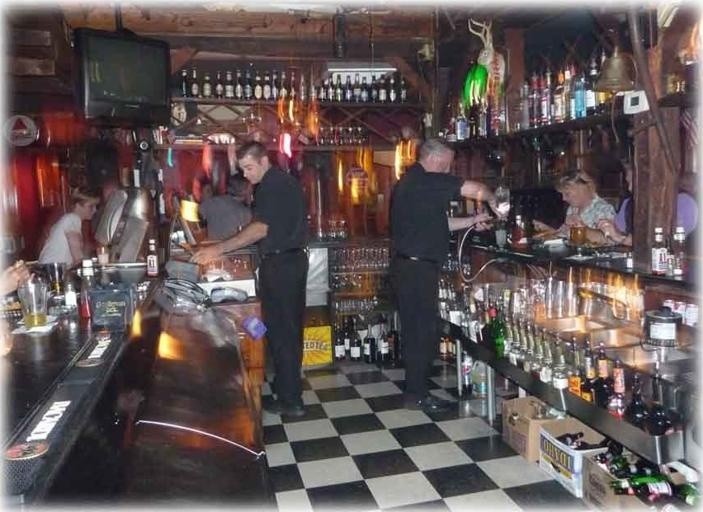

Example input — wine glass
[568,226,588,260]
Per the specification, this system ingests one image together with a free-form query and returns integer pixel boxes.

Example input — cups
[495,230,508,246]
[96,245,110,263]
[543,275,579,317]
[663,297,698,327]
[13,279,49,327]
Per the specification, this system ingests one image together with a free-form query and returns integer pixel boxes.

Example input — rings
[605,223,608,226]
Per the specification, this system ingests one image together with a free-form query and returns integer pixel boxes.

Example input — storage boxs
[294,311,333,373]
[496,393,657,512]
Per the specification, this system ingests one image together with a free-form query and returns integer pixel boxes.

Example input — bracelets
[619,232,627,245]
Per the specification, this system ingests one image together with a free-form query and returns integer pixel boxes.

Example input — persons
[385,136,508,407]
[34,185,99,267]
[188,143,306,417]
[566,156,700,257]
[199,176,255,242]
[1,260,36,297]
[531,172,618,249]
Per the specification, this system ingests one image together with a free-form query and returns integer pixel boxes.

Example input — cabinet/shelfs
[153,92,427,156]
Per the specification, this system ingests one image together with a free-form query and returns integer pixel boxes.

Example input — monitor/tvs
[72,27,172,129]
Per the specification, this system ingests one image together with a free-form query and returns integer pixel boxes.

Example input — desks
[436,267,499,399]
[461,279,701,470]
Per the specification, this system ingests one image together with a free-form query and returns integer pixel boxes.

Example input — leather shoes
[405,393,450,410]
[263,392,309,417]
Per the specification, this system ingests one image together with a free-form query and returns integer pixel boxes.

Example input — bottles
[515,215,525,230]
[335,318,398,369]
[177,63,409,103]
[144,239,158,277]
[326,219,348,241]
[80,259,95,318]
[328,247,390,272]
[444,45,619,143]
[329,274,386,291]
[495,185,511,218]
[62,280,78,309]
[652,225,688,278]
[438,281,676,437]
[336,296,381,313]
[556,433,702,512]
[314,124,367,145]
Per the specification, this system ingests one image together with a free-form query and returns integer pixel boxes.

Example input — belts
[392,248,443,270]
[259,241,309,256]
